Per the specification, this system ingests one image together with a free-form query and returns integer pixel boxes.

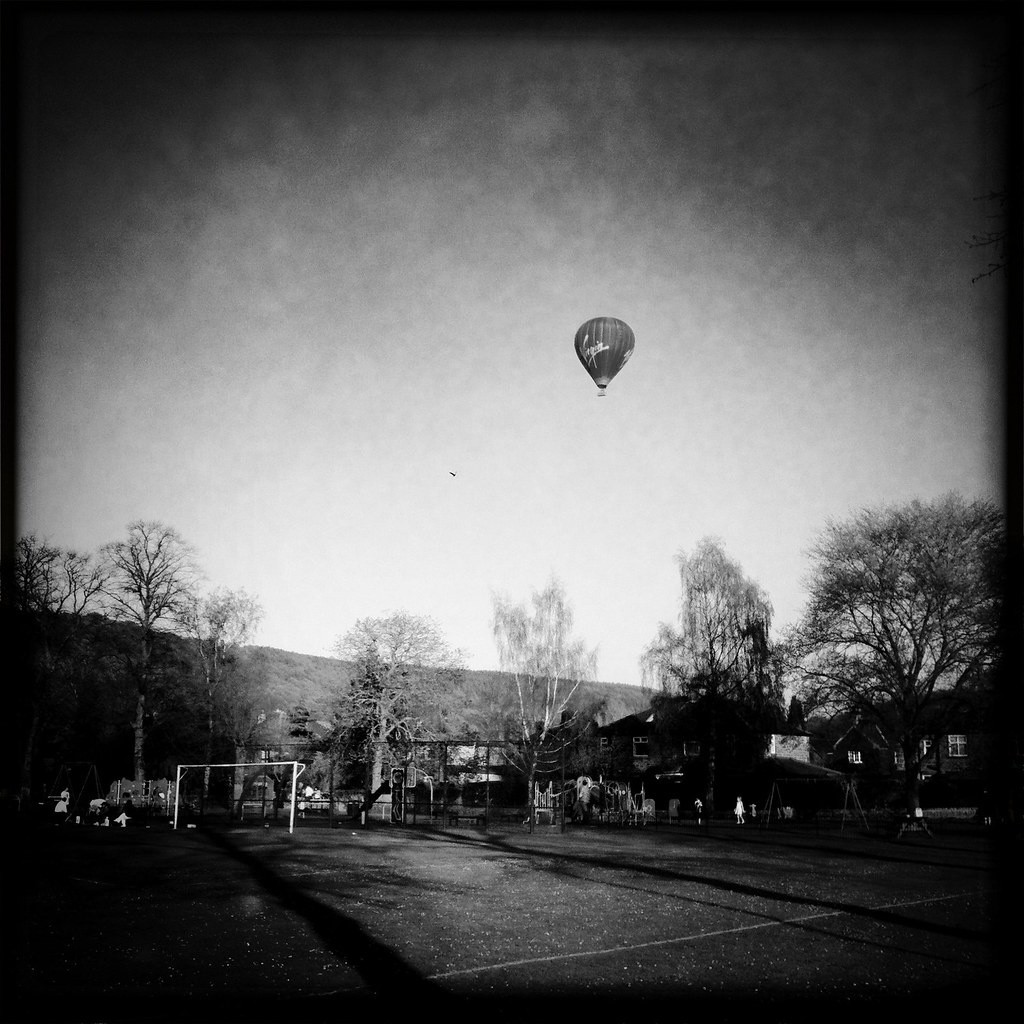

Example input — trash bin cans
[347,800,360,816]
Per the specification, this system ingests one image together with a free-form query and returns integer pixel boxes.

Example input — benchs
[449,814,486,827]
[501,815,523,823]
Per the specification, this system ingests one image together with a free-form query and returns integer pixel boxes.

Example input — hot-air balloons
[574,316,635,397]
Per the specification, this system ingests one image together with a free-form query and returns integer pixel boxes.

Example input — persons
[695,797,704,824]
[297,784,322,817]
[734,796,746,824]
[55,787,137,827]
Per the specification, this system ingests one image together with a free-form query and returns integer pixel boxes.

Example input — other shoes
[101,823,109,826]
[93,822,99,826]
[121,824,126,827]
[742,821,745,824]
[114,818,120,823]
[737,822,740,825]
